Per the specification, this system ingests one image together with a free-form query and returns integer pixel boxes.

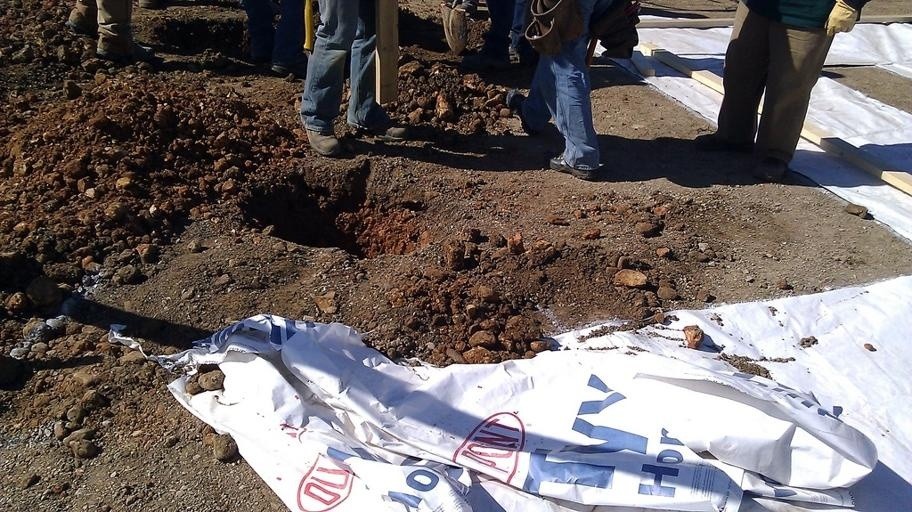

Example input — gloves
[824,0,859,35]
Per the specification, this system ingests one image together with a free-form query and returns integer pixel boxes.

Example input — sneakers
[95,43,155,62]
[507,87,546,138]
[347,110,409,142]
[299,106,339,157]
[545,150,596,181]
[695,131,787,182]
[269,58,307,80]
[67,20,97,38]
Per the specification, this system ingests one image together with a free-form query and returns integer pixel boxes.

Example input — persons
[458,1,538,74]
[506,0,602,181]
[70,0,136,65]
[692,0,871,180]
[299,0,411,156]
[241,0,308,78]
